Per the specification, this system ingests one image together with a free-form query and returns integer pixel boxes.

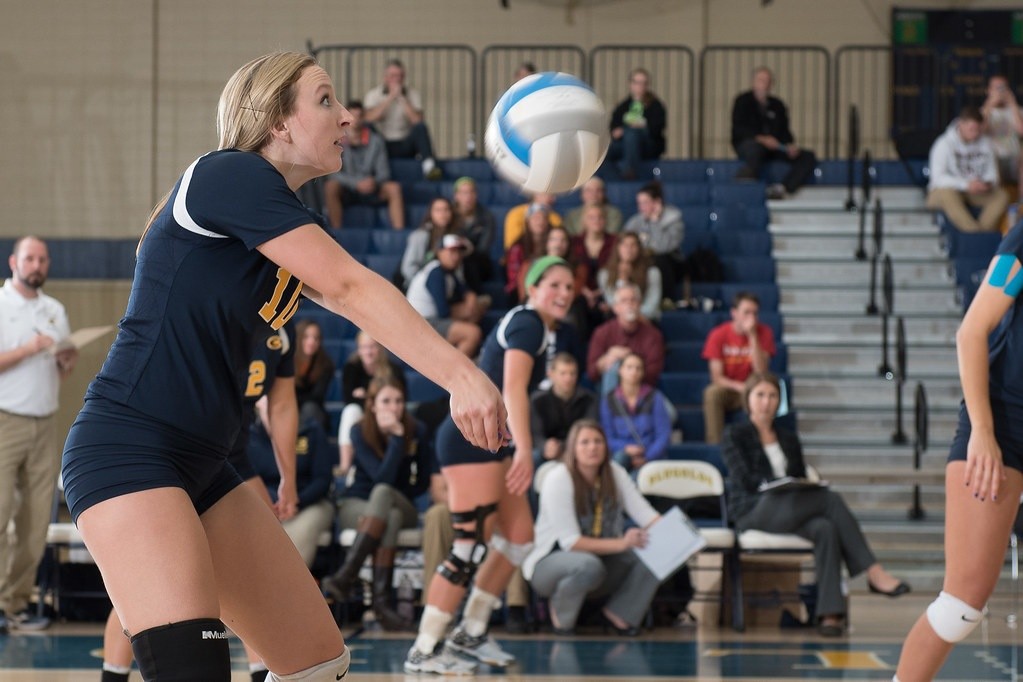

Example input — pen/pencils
[32,326,40,334]
[637,514,660,535]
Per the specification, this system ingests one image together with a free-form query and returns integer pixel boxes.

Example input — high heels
[598,607,640,637]
[546,602,576,635]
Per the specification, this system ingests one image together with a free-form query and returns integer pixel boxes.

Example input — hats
[435,233,474,256]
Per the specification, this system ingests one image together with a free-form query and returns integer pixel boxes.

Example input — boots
[371,564,410,630]
[322,530,379,606]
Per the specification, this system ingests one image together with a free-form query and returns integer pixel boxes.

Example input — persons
[61,52,511,682]
[404,254,575,676]
[248,58,707,632]
[702,290,776,445]
[730,67,817,198]
[717,373,912,639]
[102,318,301,682]
[521,419,664,636]
[0,233,79,633]
[362,59,449,182]
[925,106,1011,233]
[607,66,670,180]
[889,224,1023,681]
[978,74,1023,191]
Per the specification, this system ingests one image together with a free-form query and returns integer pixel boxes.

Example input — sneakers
[444,621,516,668]
[403,637,480,676]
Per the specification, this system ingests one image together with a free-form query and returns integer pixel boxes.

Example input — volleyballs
[484,71,612,195]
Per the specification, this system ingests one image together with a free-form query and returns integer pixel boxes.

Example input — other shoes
[821,621,843,637]
[866,575,910,598]
[0,608,51,632]
[506,604,531,635]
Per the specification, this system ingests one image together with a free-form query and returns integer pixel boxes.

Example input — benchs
[295,157,1005,478]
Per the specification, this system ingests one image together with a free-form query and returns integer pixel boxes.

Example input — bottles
[396,573,414,623]
[467,132,475,156]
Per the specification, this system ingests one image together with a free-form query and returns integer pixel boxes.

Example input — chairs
[317,460,747,634]
[718,463,821,630]
[45,467,86,625]
